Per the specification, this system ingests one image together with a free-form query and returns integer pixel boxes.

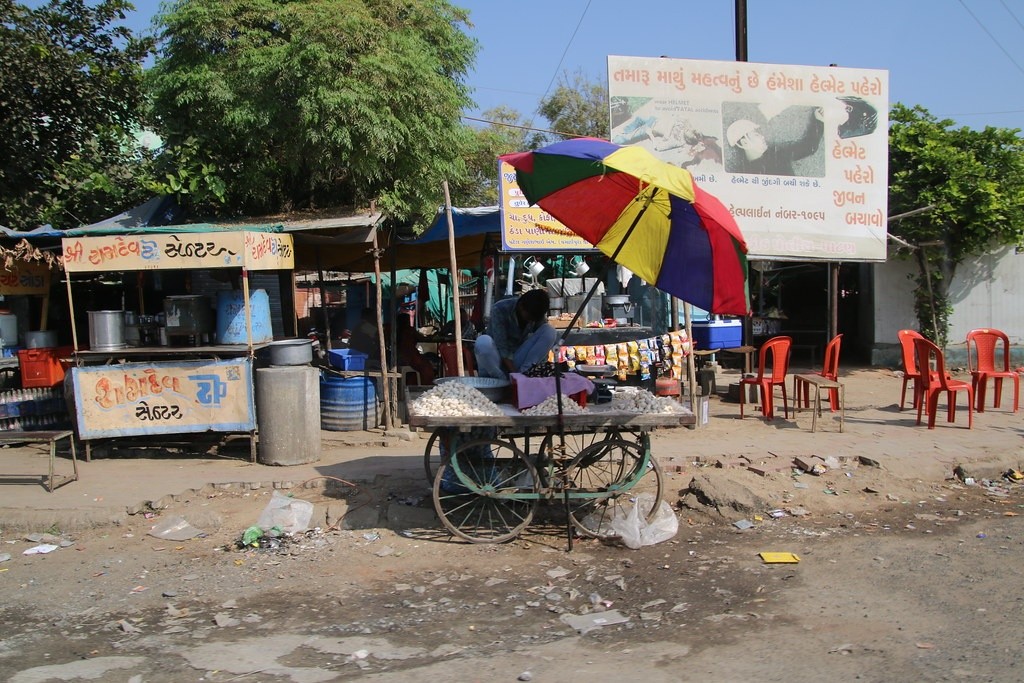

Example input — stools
[398,365,421,401]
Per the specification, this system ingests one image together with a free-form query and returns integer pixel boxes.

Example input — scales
[575,364,619,405]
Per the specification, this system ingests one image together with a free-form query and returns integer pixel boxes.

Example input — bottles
[0,387,68,431]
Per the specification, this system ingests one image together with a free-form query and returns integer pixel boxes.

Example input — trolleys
[403,377,696,544]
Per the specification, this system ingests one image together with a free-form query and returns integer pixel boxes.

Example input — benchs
[792,344,819,367]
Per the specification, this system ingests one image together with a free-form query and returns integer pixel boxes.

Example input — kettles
[522,256,545,279]
[568,256,590,277]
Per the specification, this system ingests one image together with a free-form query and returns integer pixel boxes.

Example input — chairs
[913,337,973,431]
[897,328,951,415]
[967,327,1019,414]
[740,335,793,420]
[797,333,844,413]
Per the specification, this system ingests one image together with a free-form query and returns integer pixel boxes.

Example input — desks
[779,330,827,344]
[793,374,844,432]
[417,337,476,377]
[694,347,757,373]
[0,430,79,493]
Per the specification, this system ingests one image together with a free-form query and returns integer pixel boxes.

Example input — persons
[475,289,557,379]
[612,111,703,151]
[384,313,432,385]
[727,109,824,176]
[433,309,475,363]
[499,273,507,295]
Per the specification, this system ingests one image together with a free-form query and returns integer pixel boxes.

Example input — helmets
[837,95,878,139]
[726,119,759,148]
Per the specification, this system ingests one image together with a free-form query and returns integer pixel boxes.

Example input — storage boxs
[18,345,88,388]
[326,348,369,371]
[691,318,742,350]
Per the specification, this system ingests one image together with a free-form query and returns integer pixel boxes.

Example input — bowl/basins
[269,339,314,366]
[433,376,511,403]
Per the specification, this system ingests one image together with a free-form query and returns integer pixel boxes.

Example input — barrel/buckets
[603,295,630,326]
[26,329,60,350]
[86,310,127,351]
[567,292,602,325]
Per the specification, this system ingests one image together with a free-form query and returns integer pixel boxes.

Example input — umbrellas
[498,137,754,551]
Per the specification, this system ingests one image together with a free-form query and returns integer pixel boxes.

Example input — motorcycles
[305,310,352,363]
